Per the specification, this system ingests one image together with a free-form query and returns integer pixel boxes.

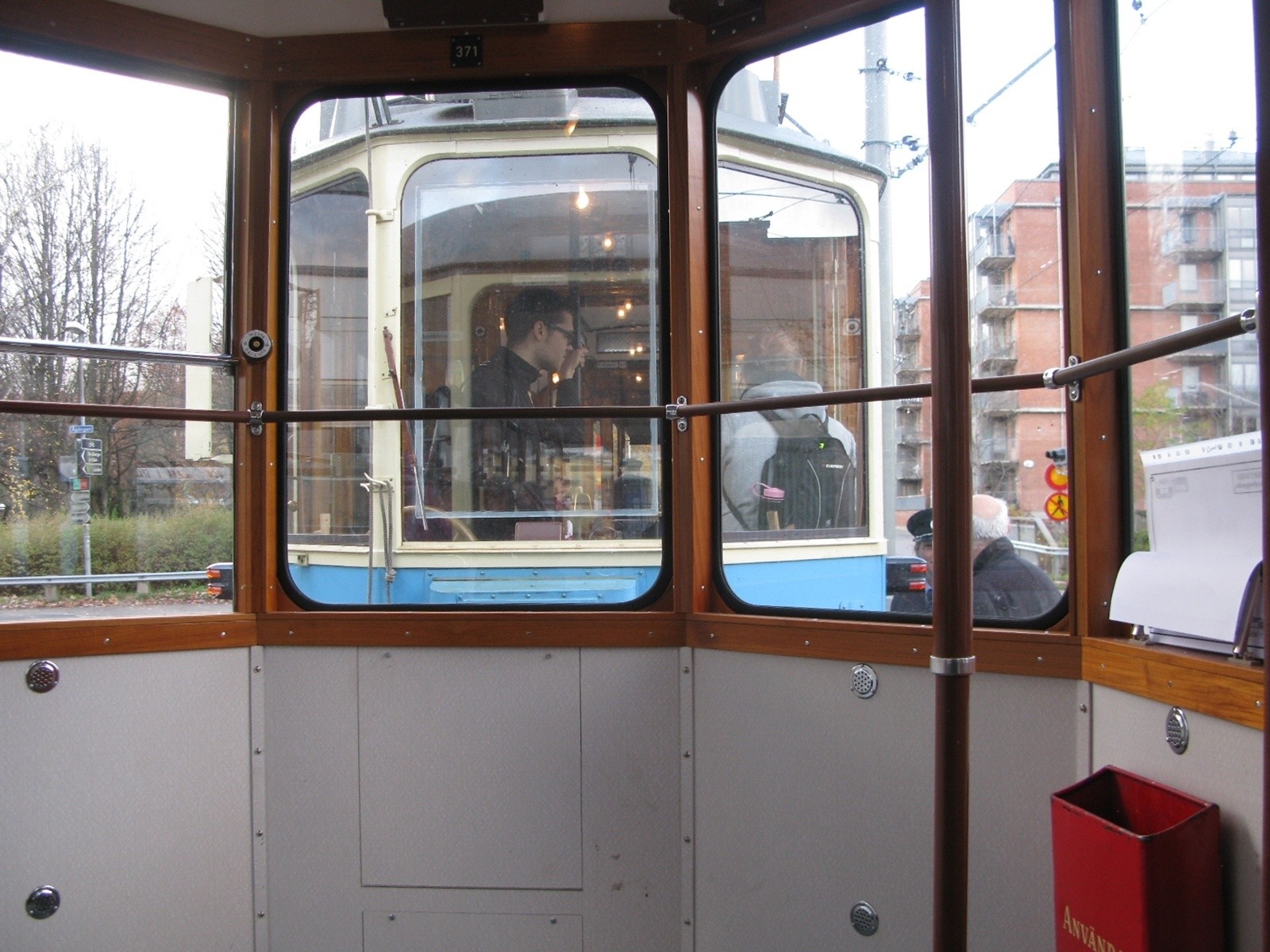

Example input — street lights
[64,321,92,600]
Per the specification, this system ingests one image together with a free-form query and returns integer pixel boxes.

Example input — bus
[205,81,927,615]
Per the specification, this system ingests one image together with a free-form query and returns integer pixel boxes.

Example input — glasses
[542,320,574,344]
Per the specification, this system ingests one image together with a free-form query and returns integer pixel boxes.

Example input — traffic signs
[70,490,90,502]
[69,512,91,524]
[81,449,103,464]
[82,464,103,475]
[70,502,90,513]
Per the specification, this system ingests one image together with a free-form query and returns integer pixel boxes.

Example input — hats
[907,508,933,541]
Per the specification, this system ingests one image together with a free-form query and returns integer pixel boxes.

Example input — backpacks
[722,409,858,530]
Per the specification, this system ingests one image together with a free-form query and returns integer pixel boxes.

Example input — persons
[971,494,1062,620]
[469,288,589,540]
[890,508,932,612]
[719,326,858,532]
